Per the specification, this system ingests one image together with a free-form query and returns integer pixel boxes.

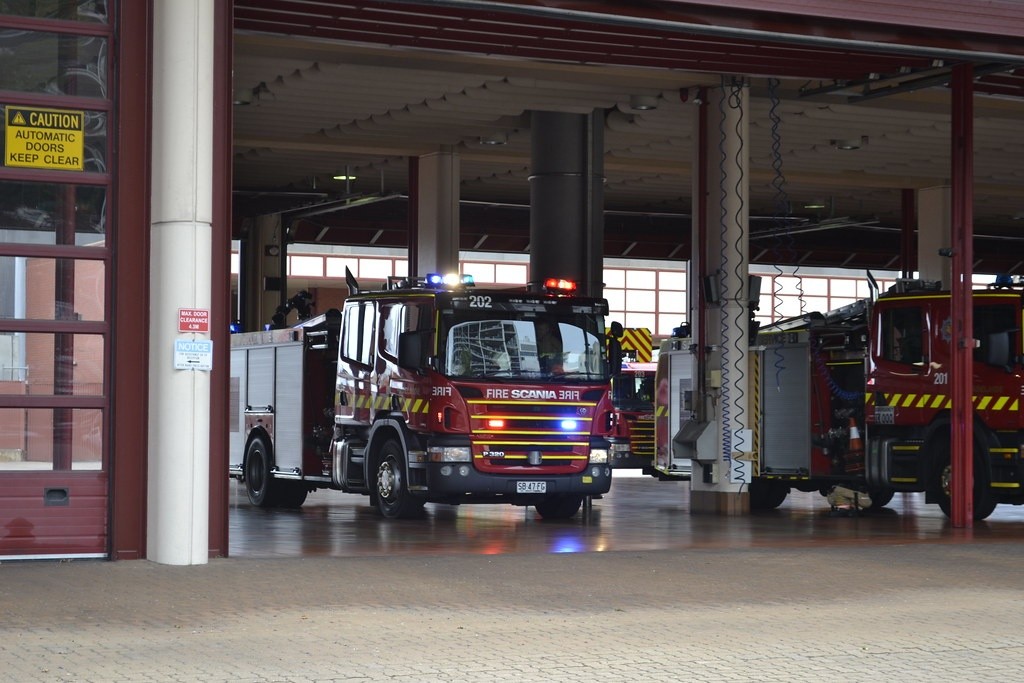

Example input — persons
[536,325,564,351]
[657,364,667,404]
[640,383,651,402]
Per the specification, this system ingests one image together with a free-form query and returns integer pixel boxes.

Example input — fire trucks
[653,276,1024,525]
[603,349,661,480]
[229,266,624,521]
